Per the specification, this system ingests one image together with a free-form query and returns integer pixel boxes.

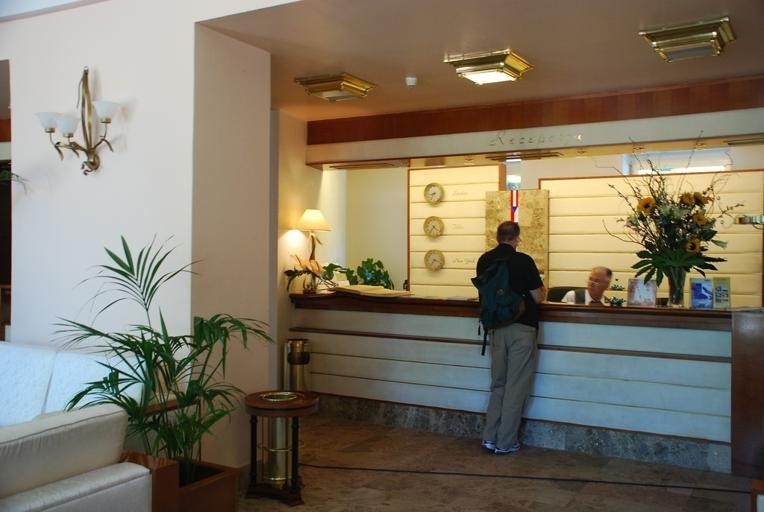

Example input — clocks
[422,216,445,238]
[423,182,444,206]
[424,249,445,273]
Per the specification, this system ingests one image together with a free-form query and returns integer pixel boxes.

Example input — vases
[665,264,685,308]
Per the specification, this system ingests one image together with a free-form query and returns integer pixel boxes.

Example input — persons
[562,266,613,306]
[476,222,544,455]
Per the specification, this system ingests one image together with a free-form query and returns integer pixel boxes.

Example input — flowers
[585,128,752,301]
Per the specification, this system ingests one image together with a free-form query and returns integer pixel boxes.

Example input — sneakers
[481,440,520,454]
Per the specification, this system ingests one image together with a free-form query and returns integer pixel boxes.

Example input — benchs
[0,402,155,512]
[0,342,155,426]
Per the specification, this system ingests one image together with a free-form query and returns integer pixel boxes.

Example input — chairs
[545,286,586,305]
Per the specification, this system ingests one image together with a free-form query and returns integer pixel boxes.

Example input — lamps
[37,63,124,179]
[637,14,732,67]
[296,206,332,271]
[294,73,373,104]
[438,49,537,86]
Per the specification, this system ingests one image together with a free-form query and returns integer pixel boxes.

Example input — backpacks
[471,256,525,330]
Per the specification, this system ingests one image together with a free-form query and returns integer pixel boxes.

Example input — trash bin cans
[283,338,312,391]
[244,389,320,507]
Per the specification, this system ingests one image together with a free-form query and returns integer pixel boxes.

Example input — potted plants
[47,232,275,512]
